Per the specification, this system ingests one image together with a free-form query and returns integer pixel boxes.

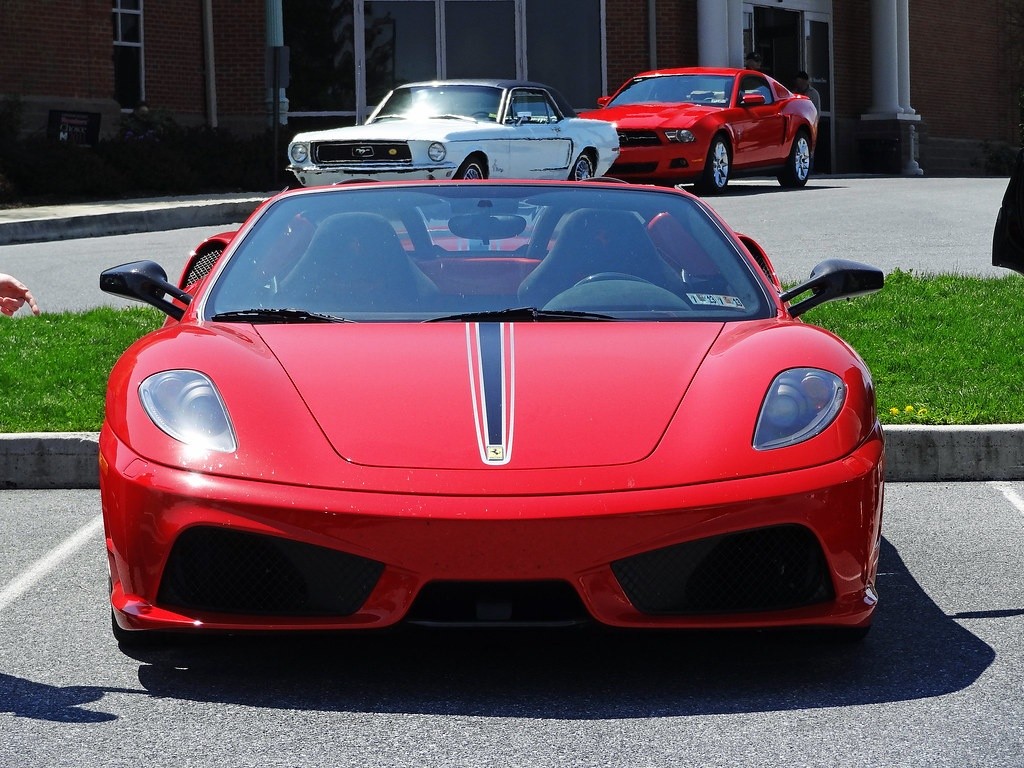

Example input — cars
[573,65,821,195]
[285,78,619,188]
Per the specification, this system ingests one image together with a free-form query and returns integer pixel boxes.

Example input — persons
[792,71,821,175]
[746,51,762,71]
[0,273,40,317]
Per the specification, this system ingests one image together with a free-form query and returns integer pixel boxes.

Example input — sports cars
[98,178,888,645]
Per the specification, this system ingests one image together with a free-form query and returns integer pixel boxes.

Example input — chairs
[515,205,681,311]
[266,212,446,311]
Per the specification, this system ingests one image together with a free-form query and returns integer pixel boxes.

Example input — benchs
[688,90,767,103]
